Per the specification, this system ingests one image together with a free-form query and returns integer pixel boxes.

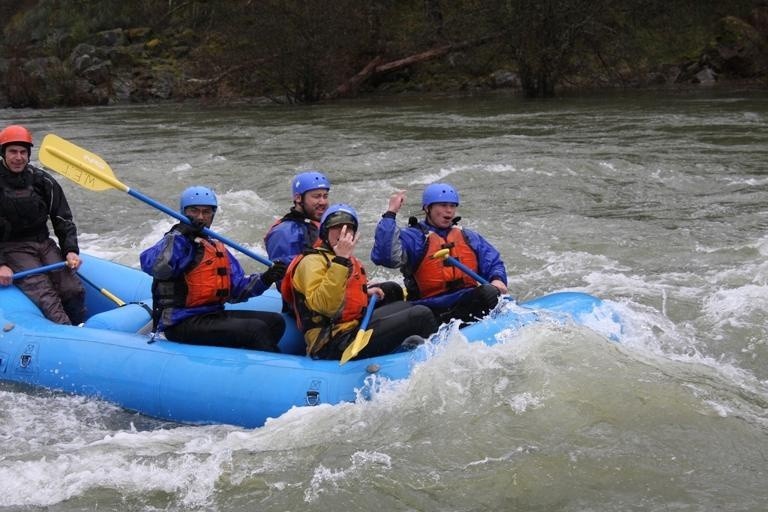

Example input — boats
[0,251,625,432]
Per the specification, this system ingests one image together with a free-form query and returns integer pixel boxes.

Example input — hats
[325,211,356,229]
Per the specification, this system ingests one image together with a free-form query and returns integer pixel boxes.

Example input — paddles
[39,134,275,267]
[339,294,377,366]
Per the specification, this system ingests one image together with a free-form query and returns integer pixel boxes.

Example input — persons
[1,125,90,329]
[265,171,403,320]
[138,185,288,355]
[371,181,509,330]
[287,203,441,362]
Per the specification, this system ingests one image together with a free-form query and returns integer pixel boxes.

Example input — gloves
[261,260,287,285]
[176,216,207,240]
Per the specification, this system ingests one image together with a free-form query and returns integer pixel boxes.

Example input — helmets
[292,172,331,201]
[180,186,218,222]
[422,183,459,210]
[318,202,359,240]
[0,125,34,146]
